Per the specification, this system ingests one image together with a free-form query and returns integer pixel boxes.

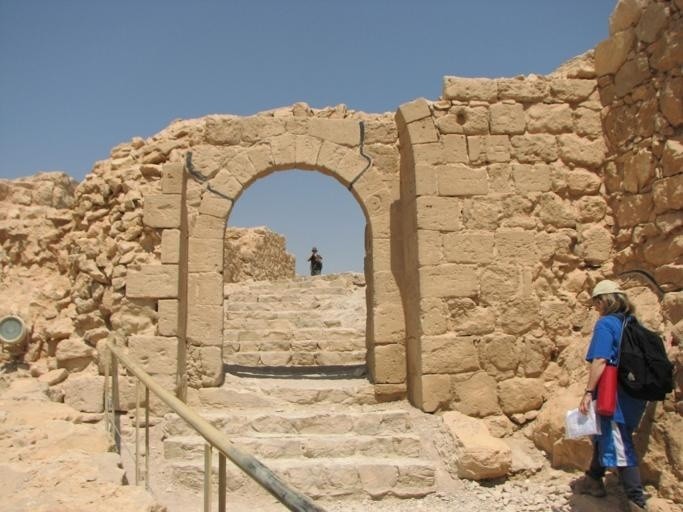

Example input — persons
[569,279,648,512]
[307,247,322,276]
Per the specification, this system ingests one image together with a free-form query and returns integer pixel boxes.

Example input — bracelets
[586,391,593,393]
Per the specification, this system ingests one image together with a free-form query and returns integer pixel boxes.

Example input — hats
[585,279,626,304]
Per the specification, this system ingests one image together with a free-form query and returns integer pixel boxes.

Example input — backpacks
[618,312,676,402]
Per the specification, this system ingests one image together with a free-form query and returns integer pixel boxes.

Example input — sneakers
[569,473,608,498]
